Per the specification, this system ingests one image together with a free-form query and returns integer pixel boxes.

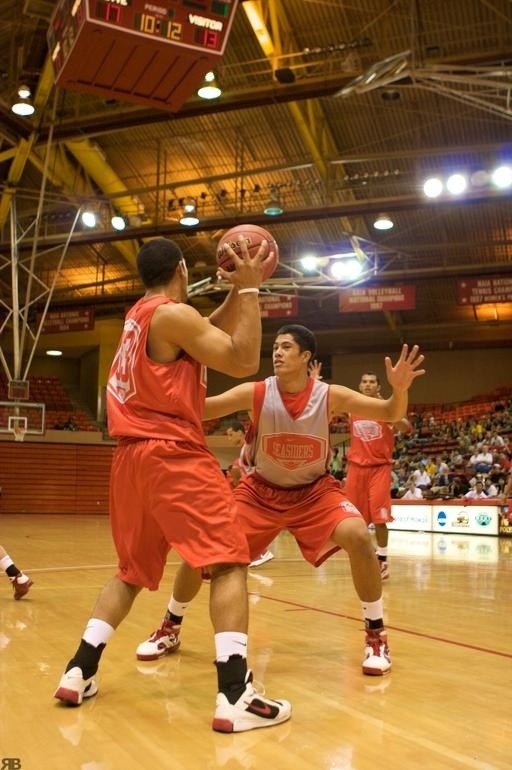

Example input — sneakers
[247,549,275,569]
[375,552,391,581]
[361,622,392,677]
[211,667,294,735]
[7,568,34,603]
[136,618,183,661]
[54,651,99,708]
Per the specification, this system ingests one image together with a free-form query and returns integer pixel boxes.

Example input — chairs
[1,372,98,431]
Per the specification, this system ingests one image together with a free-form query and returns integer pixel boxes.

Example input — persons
[328,416,351,483]
[390,400,512,500]
[226,422,274,569]
[64,417,80,431]
[52,234,293,733]
[136,324,426,676]
[306,359,413,581]
[54,417,65,430]
[0,546,33,600]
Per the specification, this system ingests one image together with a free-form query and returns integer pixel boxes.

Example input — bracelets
[238,288,259,295]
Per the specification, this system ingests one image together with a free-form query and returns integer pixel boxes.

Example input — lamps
[373,211,395,230]
[264,188,283,215]
[9,82,40,117]
[110,212,131,231]
[194,68,227,100]
[178,197,200,226]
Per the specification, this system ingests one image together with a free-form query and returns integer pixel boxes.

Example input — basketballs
[215,225,278,283]
